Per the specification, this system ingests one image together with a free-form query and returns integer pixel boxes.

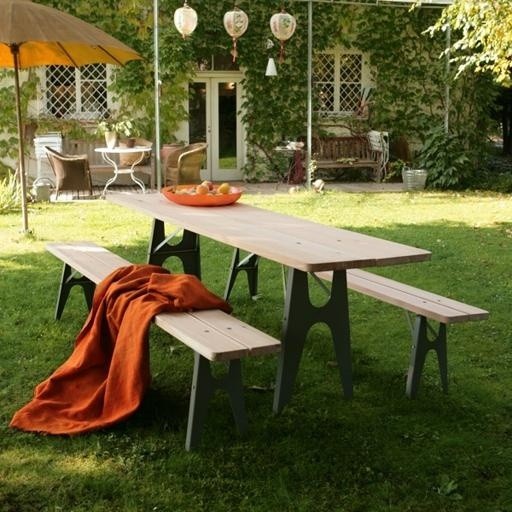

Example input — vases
[104,131,118,149]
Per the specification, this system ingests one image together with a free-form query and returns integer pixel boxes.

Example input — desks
[94,145,151,199]
[105,190,433,414]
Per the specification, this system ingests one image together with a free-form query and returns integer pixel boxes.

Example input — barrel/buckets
[402,166,428,190]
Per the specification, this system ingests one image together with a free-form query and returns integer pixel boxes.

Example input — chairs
[44,147,93,199]
[164,142,207,186]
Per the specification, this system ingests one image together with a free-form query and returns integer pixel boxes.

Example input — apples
[196,185,208,194]
[202,180,214,191]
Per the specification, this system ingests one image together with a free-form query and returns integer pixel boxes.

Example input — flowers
[94,120,134,139]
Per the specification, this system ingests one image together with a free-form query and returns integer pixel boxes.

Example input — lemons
[217,182,230,194]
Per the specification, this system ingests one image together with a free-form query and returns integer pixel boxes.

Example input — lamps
[264,40,279,77]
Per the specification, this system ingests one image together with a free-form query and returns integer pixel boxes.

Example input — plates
[161,183,243,207]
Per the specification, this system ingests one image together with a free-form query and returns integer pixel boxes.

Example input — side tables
[275,146,305,192]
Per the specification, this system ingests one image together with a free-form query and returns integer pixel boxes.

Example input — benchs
[47,242,282,454]
[224,245,490,398]
[295,137,389,182]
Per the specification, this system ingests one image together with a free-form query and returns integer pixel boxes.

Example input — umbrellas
[0,0,146,231]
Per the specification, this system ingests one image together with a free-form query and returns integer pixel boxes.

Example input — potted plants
[382,158,429,192]
[127,129,140,148]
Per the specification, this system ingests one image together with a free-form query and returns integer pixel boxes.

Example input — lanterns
[222,5,249,63]
[173,3,199,42]
[268,6,297,66]
